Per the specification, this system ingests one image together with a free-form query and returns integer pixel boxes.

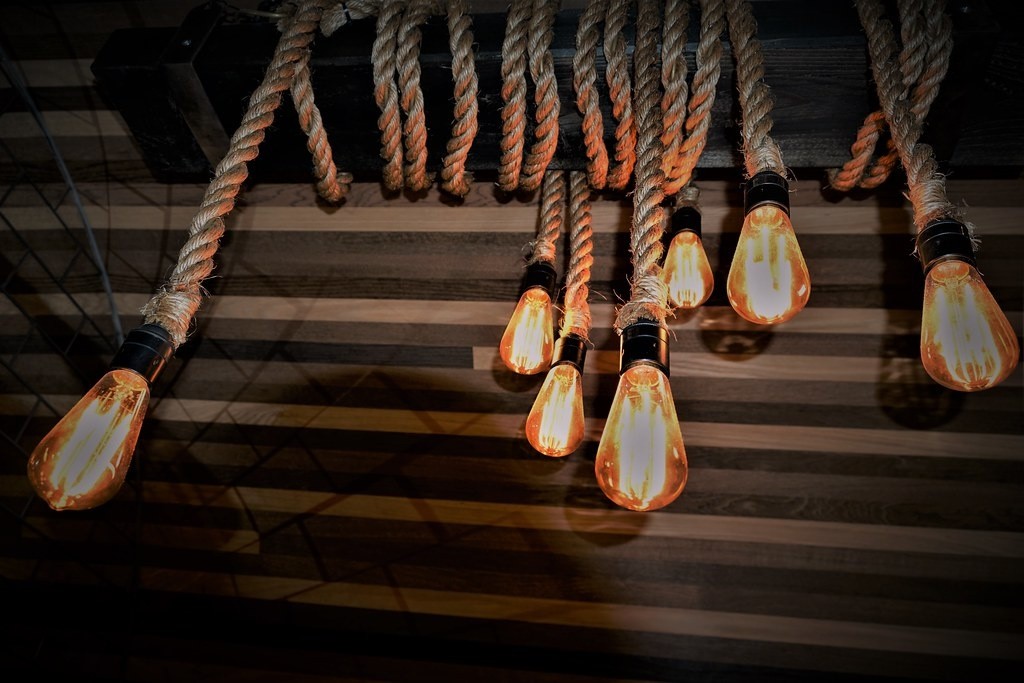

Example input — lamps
[666,170,715,307]
[853,2,1022,391]
[522,169,596,455]
[497,172,564,375]
[721,1,811,324]
[592,0,691,515]
[26,0,330,511]
